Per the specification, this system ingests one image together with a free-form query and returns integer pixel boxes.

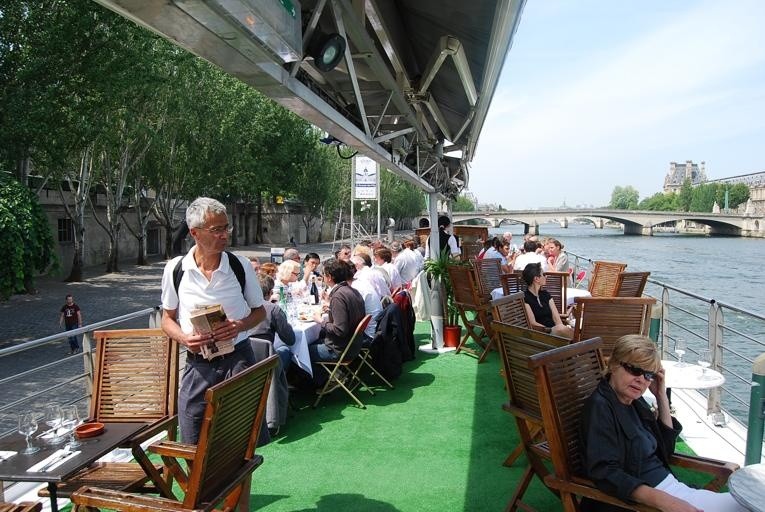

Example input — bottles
[286,288,294,324]
[278,286,286,313]
[308,277,320,305]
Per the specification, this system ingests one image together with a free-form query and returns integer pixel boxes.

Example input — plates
[297,316,314,323]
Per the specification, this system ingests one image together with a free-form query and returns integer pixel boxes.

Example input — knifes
[39,449,76,472]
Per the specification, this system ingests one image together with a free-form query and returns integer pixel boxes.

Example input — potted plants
[422,242,463,349]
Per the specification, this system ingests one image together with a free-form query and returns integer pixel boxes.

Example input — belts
[186,338,252,363]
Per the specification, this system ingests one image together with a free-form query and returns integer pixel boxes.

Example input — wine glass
[672,338,689,369]
[59,405,83,448]
[16,411,41,456]
[696,347,714,382]
[44,402,66,445]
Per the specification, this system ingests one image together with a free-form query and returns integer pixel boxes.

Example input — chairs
[313,269,427,407]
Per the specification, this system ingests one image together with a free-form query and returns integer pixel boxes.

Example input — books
[190,303,237,362]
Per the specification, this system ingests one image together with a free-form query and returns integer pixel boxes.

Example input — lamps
[321,134,359,160]
[306,23,348,73]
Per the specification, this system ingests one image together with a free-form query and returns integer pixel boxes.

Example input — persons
[246,218,431,441]
[425,216,461,287]
[58,294,84,356]
[579,333,707,512]
[517,262,572,336]
[159,196,266,511]
[479,232,570,290]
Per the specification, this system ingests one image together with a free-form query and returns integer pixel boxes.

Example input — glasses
[618,360,657,382]
[291,257,300,260]
[193,224,234,234]
[292,272,300,277]
[537,272,546,278]
